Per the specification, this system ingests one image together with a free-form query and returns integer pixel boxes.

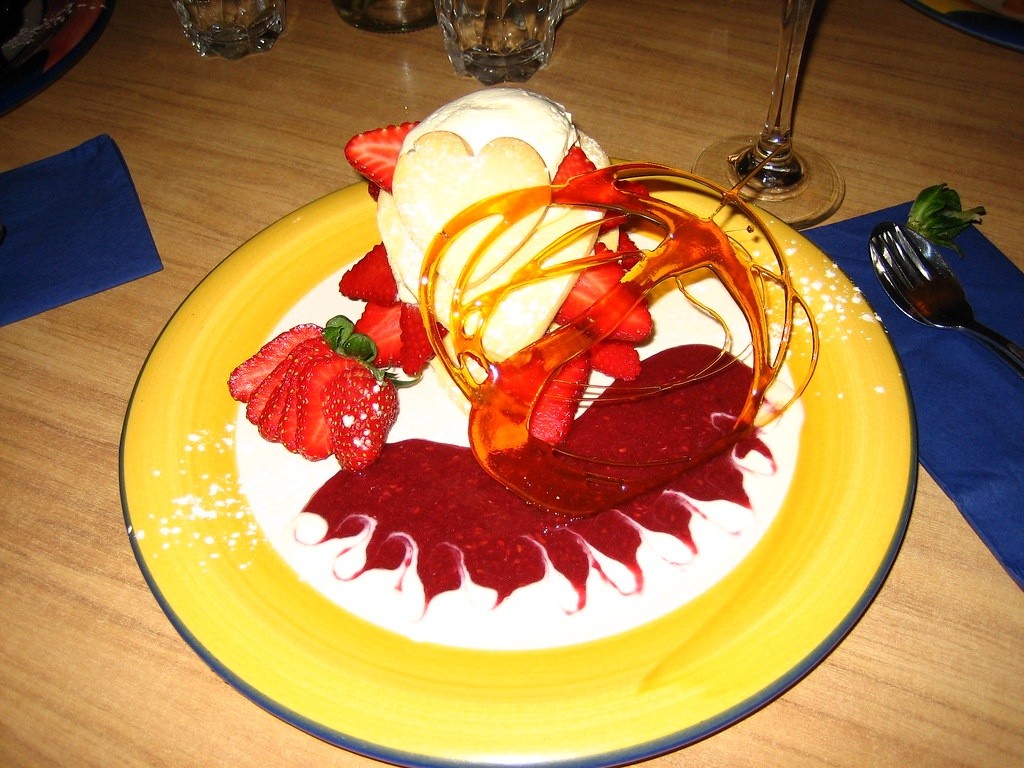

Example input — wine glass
[691,0,847,230]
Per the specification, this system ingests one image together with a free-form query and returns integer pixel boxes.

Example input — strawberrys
[228,315,395,473]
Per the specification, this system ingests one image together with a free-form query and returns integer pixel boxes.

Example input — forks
[870,226,1024,369]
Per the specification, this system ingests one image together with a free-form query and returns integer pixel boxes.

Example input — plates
[0,0,117,117]
[118,158,919,768]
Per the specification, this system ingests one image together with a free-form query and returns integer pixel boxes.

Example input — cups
[168,0,286,62]
[435,0,563,86]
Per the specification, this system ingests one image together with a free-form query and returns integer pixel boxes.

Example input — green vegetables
[906,182,987,253]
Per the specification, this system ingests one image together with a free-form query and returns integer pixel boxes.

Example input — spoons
[868,220,1024,381]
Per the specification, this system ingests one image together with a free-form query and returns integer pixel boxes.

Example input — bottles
[331,0,438,34]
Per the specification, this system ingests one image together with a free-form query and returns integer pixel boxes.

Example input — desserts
[335,86,822,517]
